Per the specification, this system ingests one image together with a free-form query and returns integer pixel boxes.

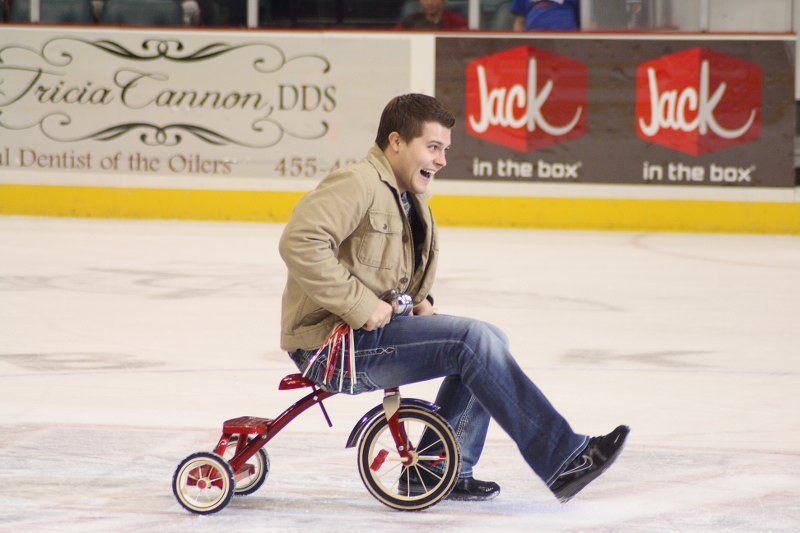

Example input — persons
[278,96,631,505]
[392,0,468,31]
[510,0,580,31]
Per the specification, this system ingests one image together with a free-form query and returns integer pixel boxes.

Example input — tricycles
[172,293,462,514]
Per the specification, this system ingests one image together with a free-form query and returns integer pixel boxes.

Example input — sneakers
[552,425,630,504]
[397,465,501,502]
